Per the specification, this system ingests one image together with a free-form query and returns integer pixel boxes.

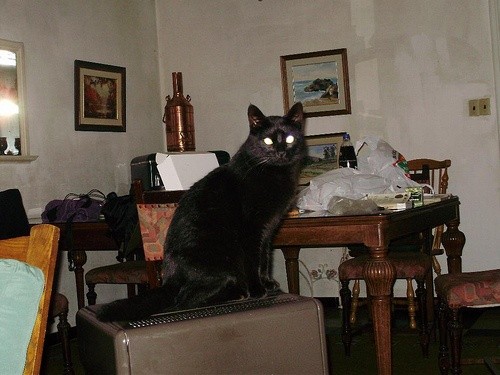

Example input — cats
[97,101,306,320]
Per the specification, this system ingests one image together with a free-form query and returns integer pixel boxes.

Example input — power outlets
[469,98,491,116]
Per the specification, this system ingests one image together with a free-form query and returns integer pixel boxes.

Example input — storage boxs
[130,151,230,190]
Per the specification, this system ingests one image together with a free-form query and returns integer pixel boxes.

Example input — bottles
[163,72,196,153]
[338,133,358,170]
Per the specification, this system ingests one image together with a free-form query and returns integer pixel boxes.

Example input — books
[367,192,412,209]
[423,194,448,202]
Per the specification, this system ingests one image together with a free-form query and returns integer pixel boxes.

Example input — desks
[271,196,465,375]
[30,221,136,310]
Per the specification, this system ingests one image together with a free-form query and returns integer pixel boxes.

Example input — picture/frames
[74,60,126,132]
[280,48,352,185]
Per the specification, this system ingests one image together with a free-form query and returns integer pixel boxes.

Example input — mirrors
[0,39,38,162]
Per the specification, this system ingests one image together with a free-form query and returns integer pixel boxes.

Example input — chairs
[0,158,500,375]
